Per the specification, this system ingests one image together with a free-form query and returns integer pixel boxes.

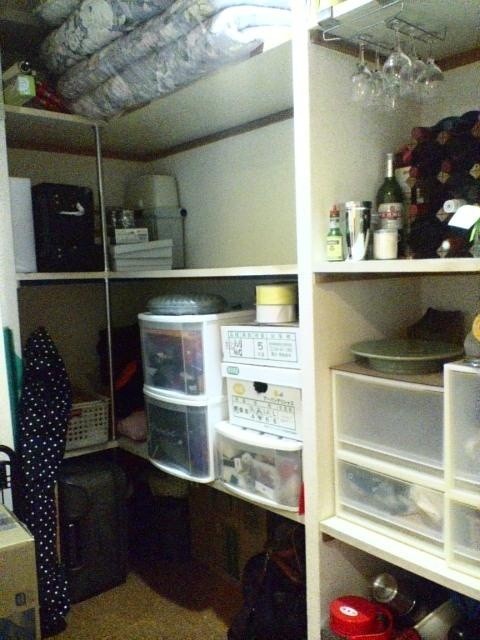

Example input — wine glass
[382,19,415,98]
[380,49,398,112]
[399,26,432,101]
[347,39,374,102]
[370,45,387,107]
[417,34,445,102]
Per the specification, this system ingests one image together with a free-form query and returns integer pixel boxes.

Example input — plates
[349,340,465,374]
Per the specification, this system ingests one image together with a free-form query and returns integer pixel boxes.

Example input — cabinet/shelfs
[1,36,306,638]
[308,0,480,639]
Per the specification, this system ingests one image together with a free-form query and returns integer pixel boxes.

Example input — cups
[375,231,399,261]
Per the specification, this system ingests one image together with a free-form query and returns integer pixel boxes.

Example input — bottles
[326,206,342,260]
[375,153,405,257]
[344,201,372,260]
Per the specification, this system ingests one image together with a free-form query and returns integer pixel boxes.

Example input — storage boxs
[444,492,480,579]
[137,309,303,513]
[334,458,447,560]
[189,481,269,628]
[444,363,480,501]
[0,503,43,639]
[331,368,444,494]
